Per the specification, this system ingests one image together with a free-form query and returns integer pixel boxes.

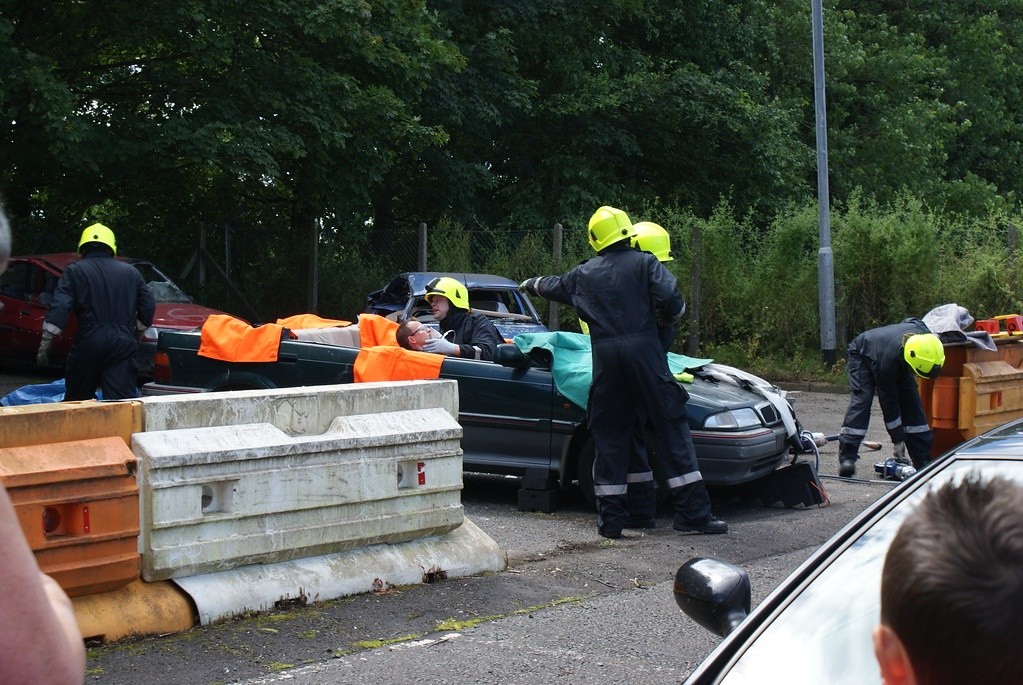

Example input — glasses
[411,325,428,336]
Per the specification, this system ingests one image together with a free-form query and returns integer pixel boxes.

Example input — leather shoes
[673,514,729,534]
[839,459,856,477]
[597,516,624,539]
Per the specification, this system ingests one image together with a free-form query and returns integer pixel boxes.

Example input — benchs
[291,324,360,347]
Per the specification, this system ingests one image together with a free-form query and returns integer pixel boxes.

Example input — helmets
[76,221,117,255]
[587,205,638,252]
[903,333,946,380]
[424,276,470,312]
[629,222,674,263]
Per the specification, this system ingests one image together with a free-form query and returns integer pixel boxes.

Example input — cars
[672,416,1023,685]
[0,252,252,386]
[367,271,550,338]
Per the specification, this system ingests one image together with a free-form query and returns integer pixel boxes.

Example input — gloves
[430,326,442,339]
[518,278,531,294]
[423,331,456,354]
[35,329,54,373]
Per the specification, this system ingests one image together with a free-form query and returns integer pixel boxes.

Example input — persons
[37,223,156,402]
[578,221,674,529]
[872,475,1023,685]
[396,317,431,352]
[838,317,946,476]
[423,277,507,361]
[0,480,87,685]
[517,206,729,539]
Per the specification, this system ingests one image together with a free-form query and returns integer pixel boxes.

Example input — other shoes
[622,518,657,529]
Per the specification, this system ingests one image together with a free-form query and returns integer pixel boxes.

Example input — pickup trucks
[154,324,803,510]
